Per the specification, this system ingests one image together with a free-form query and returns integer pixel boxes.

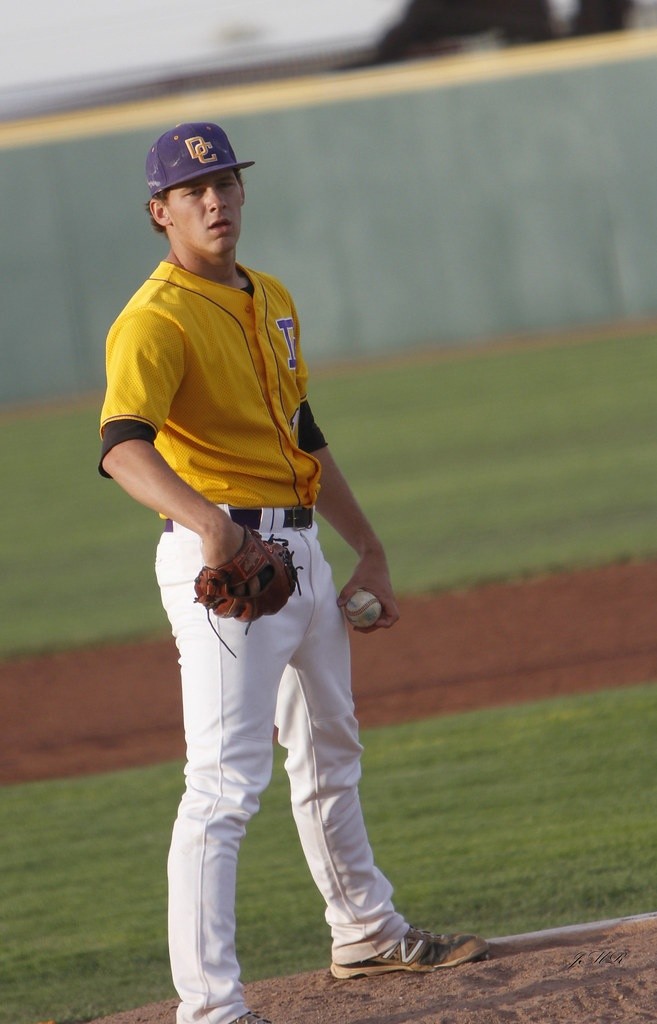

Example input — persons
[98,122,491,1024]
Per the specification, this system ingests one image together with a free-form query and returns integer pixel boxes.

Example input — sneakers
[330,925,490,979]
[229,1012,272,1024]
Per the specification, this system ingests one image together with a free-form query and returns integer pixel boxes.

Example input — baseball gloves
[194,524,298,623]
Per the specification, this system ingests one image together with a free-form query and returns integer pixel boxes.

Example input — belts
[163,506,313,531]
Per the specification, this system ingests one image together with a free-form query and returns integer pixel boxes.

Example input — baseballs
[345,590,382,628]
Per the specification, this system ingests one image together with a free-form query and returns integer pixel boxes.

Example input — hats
[146,122,256,199]
